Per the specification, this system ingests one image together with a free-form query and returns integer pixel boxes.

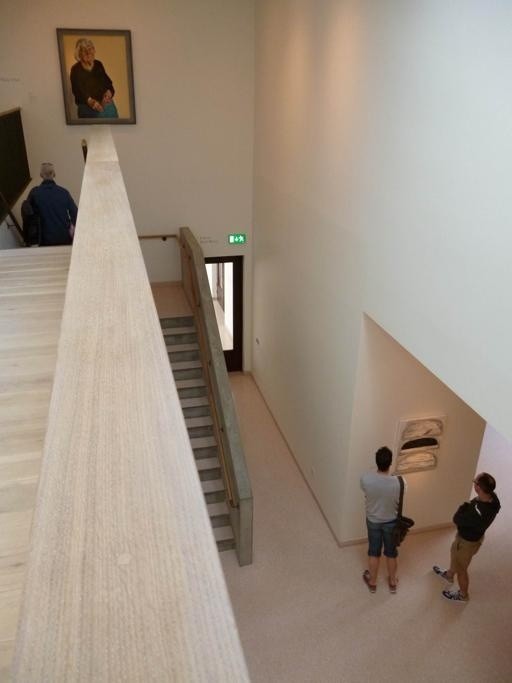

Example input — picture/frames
[56,28,136,125]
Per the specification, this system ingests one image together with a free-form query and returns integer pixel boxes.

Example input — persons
[361,447,413,593]
[22,163,77,245]
[69,38,119,118]
[433,472,503,602]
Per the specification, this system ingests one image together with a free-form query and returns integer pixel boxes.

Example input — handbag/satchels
[389,516,414,547]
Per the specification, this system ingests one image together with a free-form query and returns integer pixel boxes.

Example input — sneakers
[363,569,376,593]
[432,566,454,584]
[442,590,470,601]
[388,576,399,593]
